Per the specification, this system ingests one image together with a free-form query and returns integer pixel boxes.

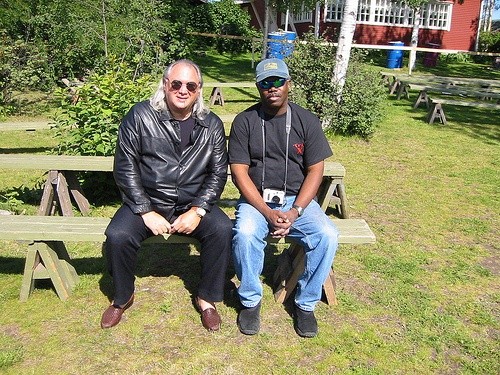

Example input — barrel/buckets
[280,31,296,58]
[387,42,404,68]
[267,33,285,60]
[423,43,440,67]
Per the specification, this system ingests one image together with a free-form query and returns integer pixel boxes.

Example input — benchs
[381,71,500,126]
[0,215,376,306]
[202,82,255,106]
[0,156,349,218]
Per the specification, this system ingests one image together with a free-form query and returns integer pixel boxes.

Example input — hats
[256,58,290,82]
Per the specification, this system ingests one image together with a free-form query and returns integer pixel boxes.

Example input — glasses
[171,80,198,91]
[255,78,286,89]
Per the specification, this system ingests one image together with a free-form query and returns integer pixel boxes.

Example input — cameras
[263,189,285,204]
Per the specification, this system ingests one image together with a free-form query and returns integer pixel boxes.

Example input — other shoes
[194,294,222,330]
[237,297,262,335]
[293,296,318,337]
[100,292,134,329]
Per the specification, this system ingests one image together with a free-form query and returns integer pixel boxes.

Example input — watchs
[190,206,207,218]
[293,205,304,216]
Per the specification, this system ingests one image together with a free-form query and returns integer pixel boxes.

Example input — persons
[100,59,234,332]
[227,58,339,338]
